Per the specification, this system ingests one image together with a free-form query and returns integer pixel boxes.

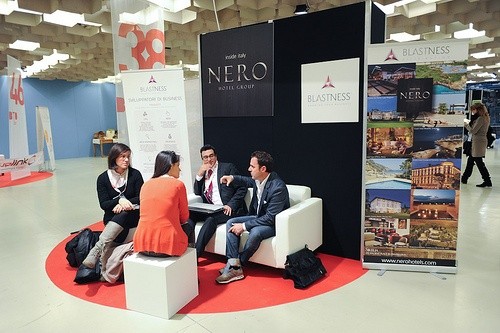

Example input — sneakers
[215,259,244,284]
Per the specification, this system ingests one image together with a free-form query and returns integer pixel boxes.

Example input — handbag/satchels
[284,244,327,288]
[73,259,100,283]
[463,141,472,156]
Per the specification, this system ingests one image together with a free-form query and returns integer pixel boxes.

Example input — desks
[93,139,118,158]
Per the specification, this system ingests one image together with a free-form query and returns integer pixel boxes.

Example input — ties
[205,169,212,203]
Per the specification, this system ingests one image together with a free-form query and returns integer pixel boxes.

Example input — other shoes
[476,180,492,187]
[461,177,467,184]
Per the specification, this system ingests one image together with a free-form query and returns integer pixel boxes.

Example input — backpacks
[65,228,100,267]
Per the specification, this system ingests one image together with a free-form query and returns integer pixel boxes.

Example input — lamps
[293,4,310,14]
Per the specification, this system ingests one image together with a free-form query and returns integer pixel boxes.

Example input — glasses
[202,154,215,160]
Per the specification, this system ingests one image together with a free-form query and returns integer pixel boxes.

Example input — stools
[122,247,199,319]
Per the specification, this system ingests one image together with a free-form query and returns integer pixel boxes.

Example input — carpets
[45,220,369,313]
[0,171,53,188]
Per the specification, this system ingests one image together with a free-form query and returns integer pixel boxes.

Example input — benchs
[188,185,322,274]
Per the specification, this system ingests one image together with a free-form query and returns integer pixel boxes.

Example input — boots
[82,220,124,269]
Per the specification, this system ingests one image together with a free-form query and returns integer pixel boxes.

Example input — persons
[133,150,199,285]
[82,143,144,283]
[188,144,249,265]
[216,151,290,284]
[461,102,492,187]
